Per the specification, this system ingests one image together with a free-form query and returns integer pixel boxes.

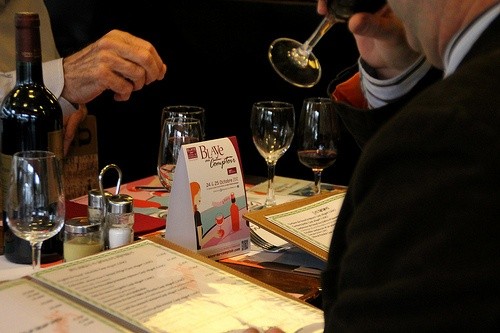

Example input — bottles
[0,11,65,264]
[106,195,135,249]
[87,190,113,220]
[63,216,104,262]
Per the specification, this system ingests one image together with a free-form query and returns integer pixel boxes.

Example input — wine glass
[156,117,207,220]
[268,0,388,88]
[298,98,343,195]
[247,101,295,212]
[6,151,65,271]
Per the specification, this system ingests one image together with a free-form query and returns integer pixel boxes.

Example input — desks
[0,174,323,333]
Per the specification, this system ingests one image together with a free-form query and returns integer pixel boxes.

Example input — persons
[317,0,500,333]
[0,0,167,213]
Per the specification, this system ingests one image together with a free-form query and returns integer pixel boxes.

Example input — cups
[161,105,205,124]
